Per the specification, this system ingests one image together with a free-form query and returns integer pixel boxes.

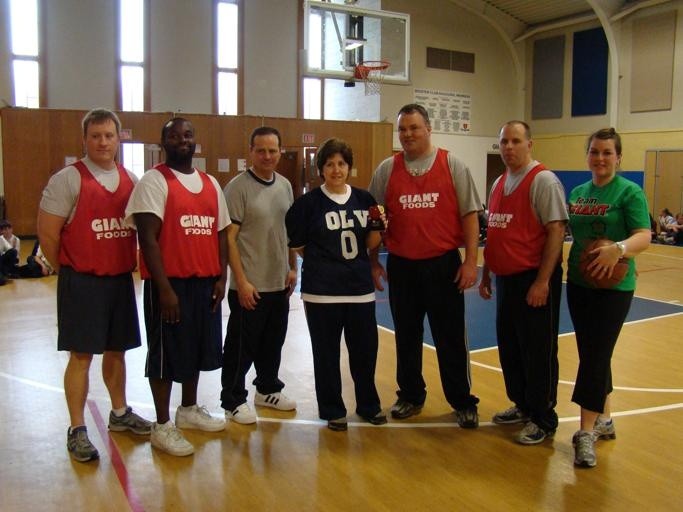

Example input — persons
[221,127,299,426]
[37,108,154,464]
[124,117,232,458]
[479,120,571,447]
[25,242,54,278]
[0,220,20,278]
[649,208,683,245]
[286,138,388,432]
[366,105,483,429]
[566,127,652,468]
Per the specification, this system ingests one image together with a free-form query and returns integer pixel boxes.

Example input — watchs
[614,241,625,257]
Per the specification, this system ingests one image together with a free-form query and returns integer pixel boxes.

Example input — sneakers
[572,418,617,444]
[493,405,533,425]
[107,405,154,435]
[455,407,479,428]
[517,421,557,445]
[66,423,101,463]
[150,419,196,458]
[174,404,226,432]
[370,410,388,424]
[390,399,424,417]
[327,416,348,431]
[573,431,598,467]
[225,402,258,425]
[253,387,297,411]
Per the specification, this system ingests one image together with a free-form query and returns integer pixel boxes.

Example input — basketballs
[581,238,629,290]
